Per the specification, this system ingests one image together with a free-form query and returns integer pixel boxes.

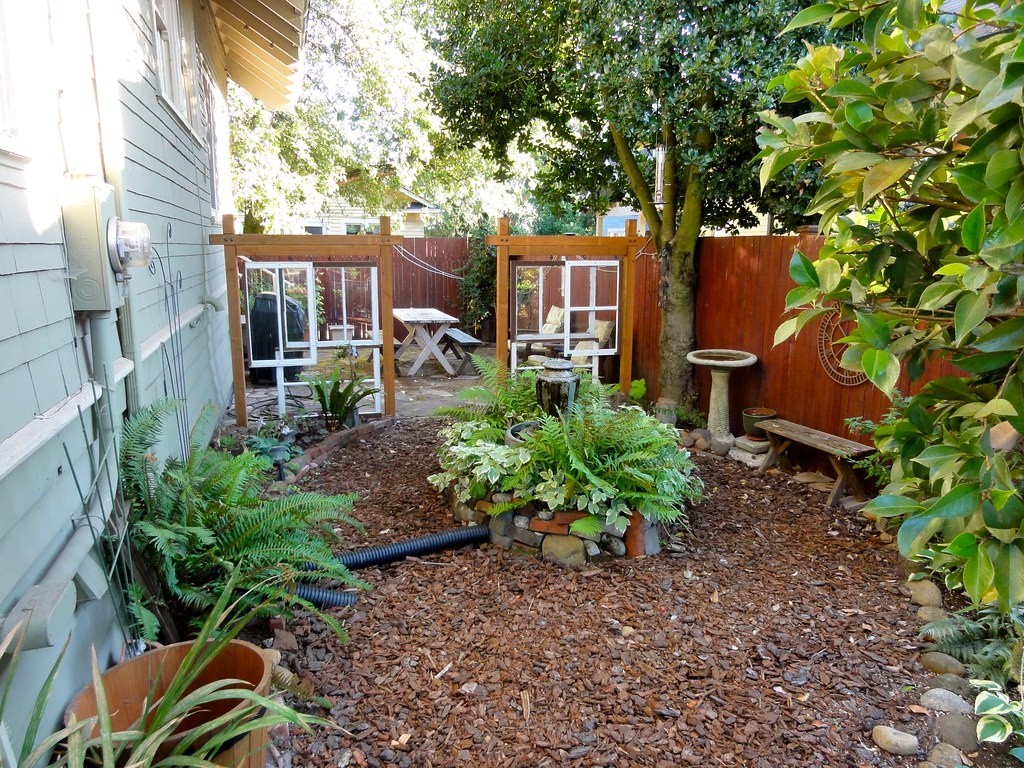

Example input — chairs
[524,319,615,384]
[505,305,565,368]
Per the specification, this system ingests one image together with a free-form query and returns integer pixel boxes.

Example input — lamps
[107,216,153,272]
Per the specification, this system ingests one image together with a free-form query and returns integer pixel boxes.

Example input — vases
[63,636,272,768]
[534,358,579,435]
[505,417,562,448]
[742,407,777,442]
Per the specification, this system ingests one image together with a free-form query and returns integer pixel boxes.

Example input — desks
[392,307,460,377]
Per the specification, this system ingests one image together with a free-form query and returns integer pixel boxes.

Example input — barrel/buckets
[62,634,270,767]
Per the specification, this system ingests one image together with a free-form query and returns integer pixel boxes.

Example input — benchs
[339,320,368,340]
[753,417,876,508]
[441,328,482,376]
[366,329,403,378]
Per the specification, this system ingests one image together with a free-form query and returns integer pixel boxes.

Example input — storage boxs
[329,324,355,341]
